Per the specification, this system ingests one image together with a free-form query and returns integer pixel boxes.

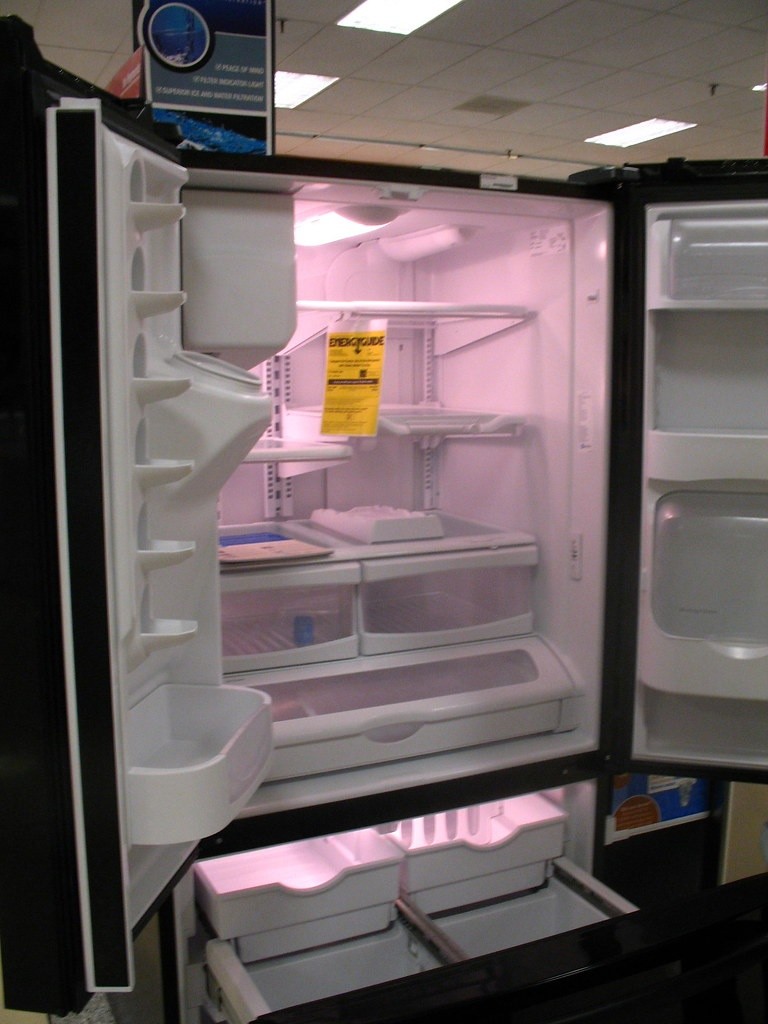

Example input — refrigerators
[0,16,768,1024]
